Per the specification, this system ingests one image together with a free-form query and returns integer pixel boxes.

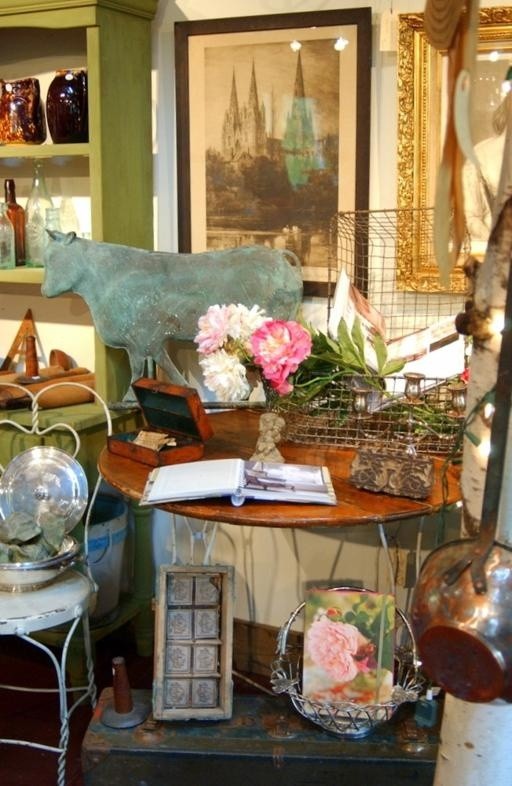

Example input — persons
[249,413,285,463]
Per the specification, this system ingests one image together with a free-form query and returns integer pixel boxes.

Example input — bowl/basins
[49,348,69,370]
[1,533,80,594]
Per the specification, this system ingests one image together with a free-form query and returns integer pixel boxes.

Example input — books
[138,457,340,507]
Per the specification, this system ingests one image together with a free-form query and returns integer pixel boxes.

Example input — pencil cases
[107,378,214,468]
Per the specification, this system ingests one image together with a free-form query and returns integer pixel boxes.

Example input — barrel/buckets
[45,490,130,633]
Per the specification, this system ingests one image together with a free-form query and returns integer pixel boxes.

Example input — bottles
[46,68,89,144]
[0,157,66,271]
[0,78,46,145]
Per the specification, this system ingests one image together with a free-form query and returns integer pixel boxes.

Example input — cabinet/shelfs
[0,1,156,705]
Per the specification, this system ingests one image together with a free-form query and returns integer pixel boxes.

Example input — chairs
[0,381,112,785]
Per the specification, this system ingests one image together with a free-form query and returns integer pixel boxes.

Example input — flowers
[193,304,454,434]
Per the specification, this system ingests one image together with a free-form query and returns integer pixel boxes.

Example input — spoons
[16,369,87,385]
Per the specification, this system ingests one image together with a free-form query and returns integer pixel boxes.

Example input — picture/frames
[174,8,372,299]
[396,6,512,292]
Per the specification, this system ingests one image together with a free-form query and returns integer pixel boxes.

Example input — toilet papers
[432,690,512,785]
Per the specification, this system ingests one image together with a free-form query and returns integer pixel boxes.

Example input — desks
[96,408,464,697]
